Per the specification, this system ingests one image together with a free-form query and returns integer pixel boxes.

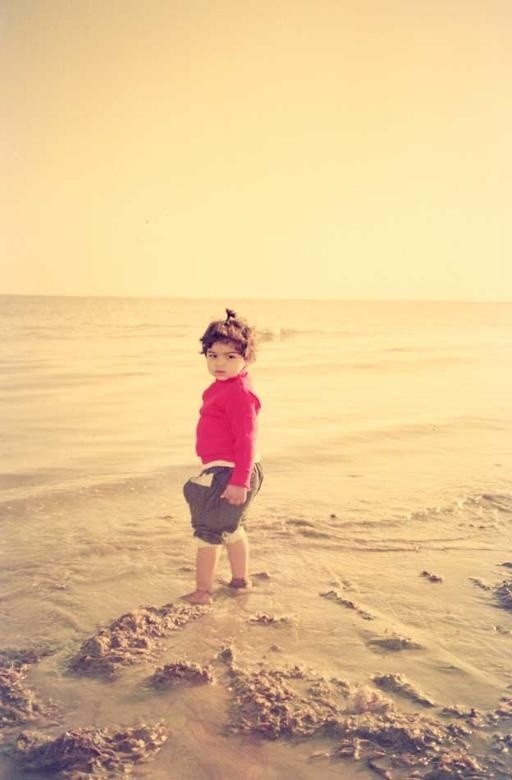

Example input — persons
[178,307,265,605]
